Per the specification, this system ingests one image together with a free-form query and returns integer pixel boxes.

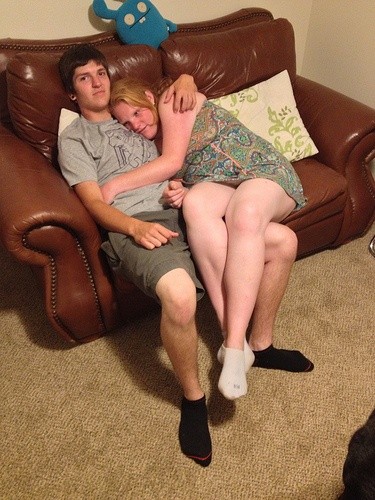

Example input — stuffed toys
[93,0,177,52]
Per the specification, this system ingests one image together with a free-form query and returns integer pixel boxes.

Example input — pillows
[206,69,321,164]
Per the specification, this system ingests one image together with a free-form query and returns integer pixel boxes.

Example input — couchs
[0,6,375,348]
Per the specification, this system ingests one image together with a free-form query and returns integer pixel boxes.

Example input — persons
[56,44,314,467]
[100,73,304,398]
